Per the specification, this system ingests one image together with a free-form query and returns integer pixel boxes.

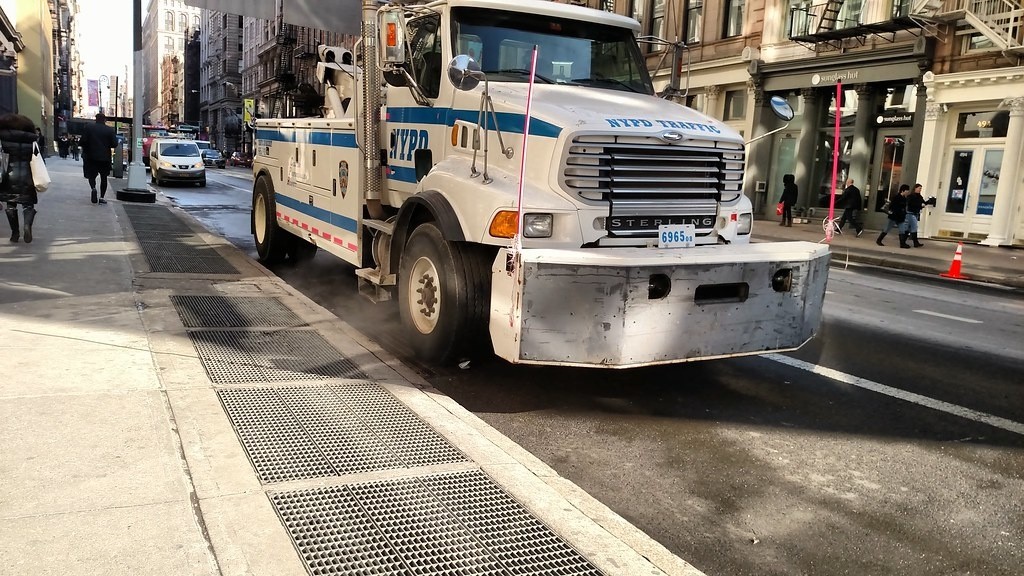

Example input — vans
[194,139,210,156]
[149,138,208,187]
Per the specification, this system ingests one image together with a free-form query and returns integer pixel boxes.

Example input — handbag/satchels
[775,202,784,216]
[30,142,51,192]
[0,141,9,184]
[880,195,902,215]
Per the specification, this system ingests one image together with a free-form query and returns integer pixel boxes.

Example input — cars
[230,151,250,167]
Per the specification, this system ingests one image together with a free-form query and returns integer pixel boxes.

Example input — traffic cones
[940,239,970,280]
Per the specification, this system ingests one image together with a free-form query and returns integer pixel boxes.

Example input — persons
[57,134,80,161]
[834,179,865,238]
[36,128,46,164]
[80,113,118,206]
[904,184,927,247]
[0,113,40,244]
[875,185,911,249]
[777,174,798,227]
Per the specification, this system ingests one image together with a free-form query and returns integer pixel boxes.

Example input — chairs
[524,44,565,75]
[419,51,442,99]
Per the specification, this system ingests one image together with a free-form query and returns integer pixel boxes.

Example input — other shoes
[99,199,107,205]
[91,189,98,203]
[856,229,863,238]
[834,229,841,235]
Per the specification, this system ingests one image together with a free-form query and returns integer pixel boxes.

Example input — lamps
[943,103,948,113]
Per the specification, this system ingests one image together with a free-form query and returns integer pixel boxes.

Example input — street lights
[98,74,110,111]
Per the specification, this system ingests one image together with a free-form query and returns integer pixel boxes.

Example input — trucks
[142,125,170,165]
[248,0,835,372]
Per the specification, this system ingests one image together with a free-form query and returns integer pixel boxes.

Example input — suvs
[201,150,227,169]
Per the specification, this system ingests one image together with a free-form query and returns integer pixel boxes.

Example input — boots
[5,209,20,242]
[785,221,792,227]
[899,234,910,248]
[876,232,886,246]
[911,232,924,247]
[779,219,785,226]
[24,208,37,243]
